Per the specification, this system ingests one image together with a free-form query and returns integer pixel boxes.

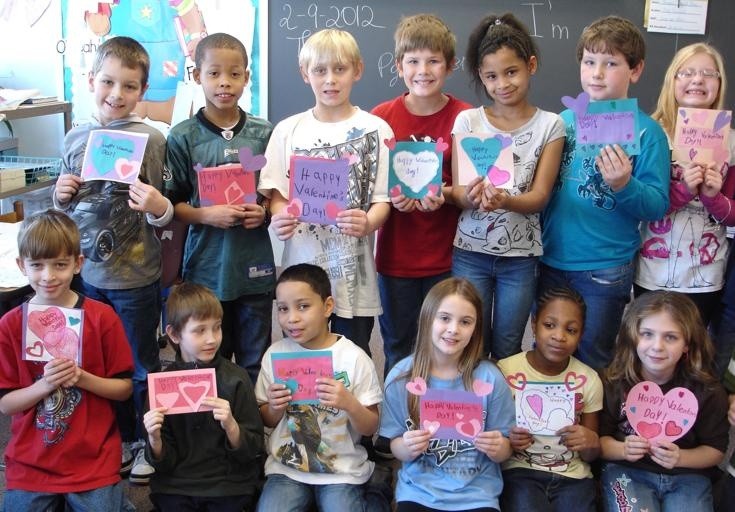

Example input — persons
[1,209,135,512]
[140,281,264,512]
[370,14,475,382]
[52,35,174,484]
[450,15,567,365]
[592,291,734,512]
[379,276,517,512]
[496,285,603,512]
[632,44,734,383]
[163,32,277,385]
[257,28,395,463]
[254,264,393,512]
[530,15,671,374]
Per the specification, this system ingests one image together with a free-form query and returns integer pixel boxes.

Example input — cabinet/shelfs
[0,99,74,200]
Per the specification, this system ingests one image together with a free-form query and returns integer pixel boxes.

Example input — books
[2,164,52,192]
[22,96,58,104]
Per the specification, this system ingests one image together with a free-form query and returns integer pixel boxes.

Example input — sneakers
[129,440,156,485]
[116,440,135,475]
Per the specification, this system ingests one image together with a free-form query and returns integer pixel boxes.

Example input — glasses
[675,68,722,80]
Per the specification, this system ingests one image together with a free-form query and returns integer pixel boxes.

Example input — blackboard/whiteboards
[268,0,734,239]
